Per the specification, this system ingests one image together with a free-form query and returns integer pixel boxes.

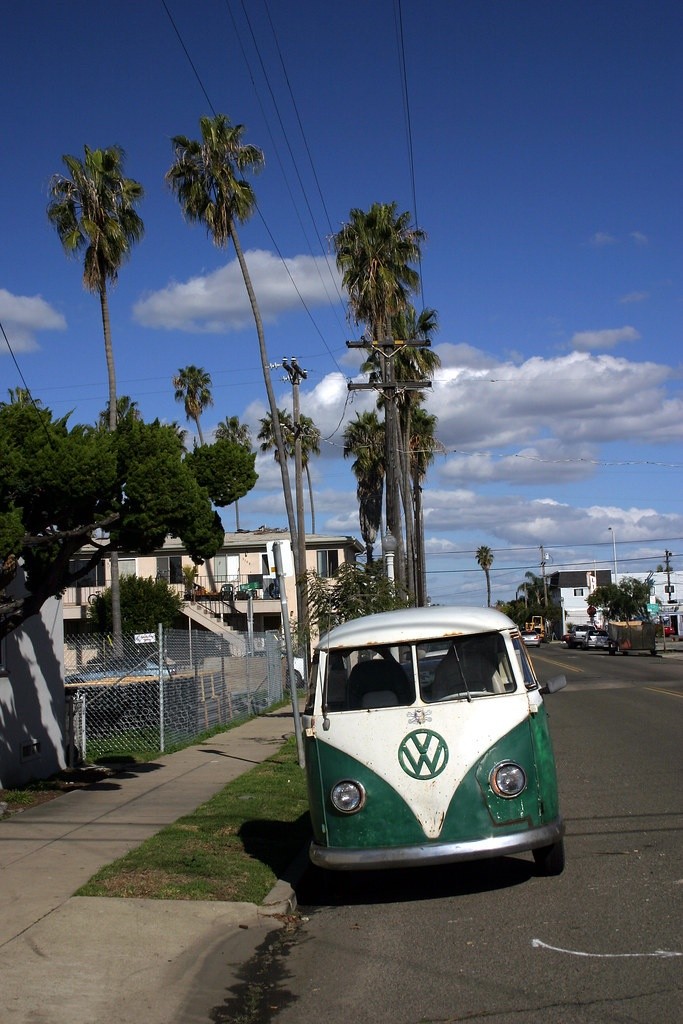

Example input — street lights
[607,527,619,586]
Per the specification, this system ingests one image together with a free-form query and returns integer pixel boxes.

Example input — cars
[520,630,542,649]
[64,650,178,688]
[243,651,306,689]
[654,622,675,636]
[293,607,567,885]
[582,629,612,651]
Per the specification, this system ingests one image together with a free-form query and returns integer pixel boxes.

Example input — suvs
[568,624,599,649]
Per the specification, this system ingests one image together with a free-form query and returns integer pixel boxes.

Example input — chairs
[430,652,508,701]
[221,583,235,602]
[347,660,412,711]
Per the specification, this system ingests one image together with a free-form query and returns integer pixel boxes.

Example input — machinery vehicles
[525,615,552,644]
